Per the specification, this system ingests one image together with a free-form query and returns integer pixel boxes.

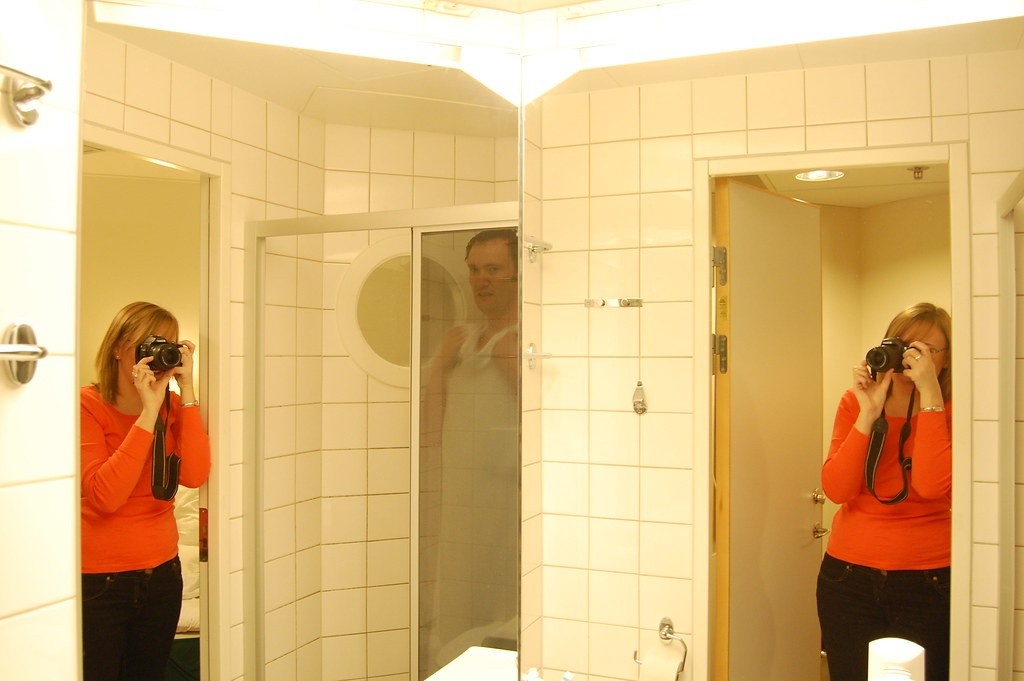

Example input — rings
[131,371,138,377]
[915,353,922,359]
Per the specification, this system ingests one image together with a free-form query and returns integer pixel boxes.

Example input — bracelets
[922,405,944,412]
[180,400,199,407]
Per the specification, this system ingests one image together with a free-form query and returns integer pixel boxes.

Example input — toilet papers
[866,636,925,681]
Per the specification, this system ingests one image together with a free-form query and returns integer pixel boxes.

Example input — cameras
[866,337,913,373]
[136,336,184,372]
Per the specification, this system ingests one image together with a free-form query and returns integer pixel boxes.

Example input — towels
[420,319,520,674]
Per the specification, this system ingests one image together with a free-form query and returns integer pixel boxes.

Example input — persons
[429,227,522,655]
[816,301,952,681]
[81,299,213,681]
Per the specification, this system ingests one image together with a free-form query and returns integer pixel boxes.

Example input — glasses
[929,345,947,362]
